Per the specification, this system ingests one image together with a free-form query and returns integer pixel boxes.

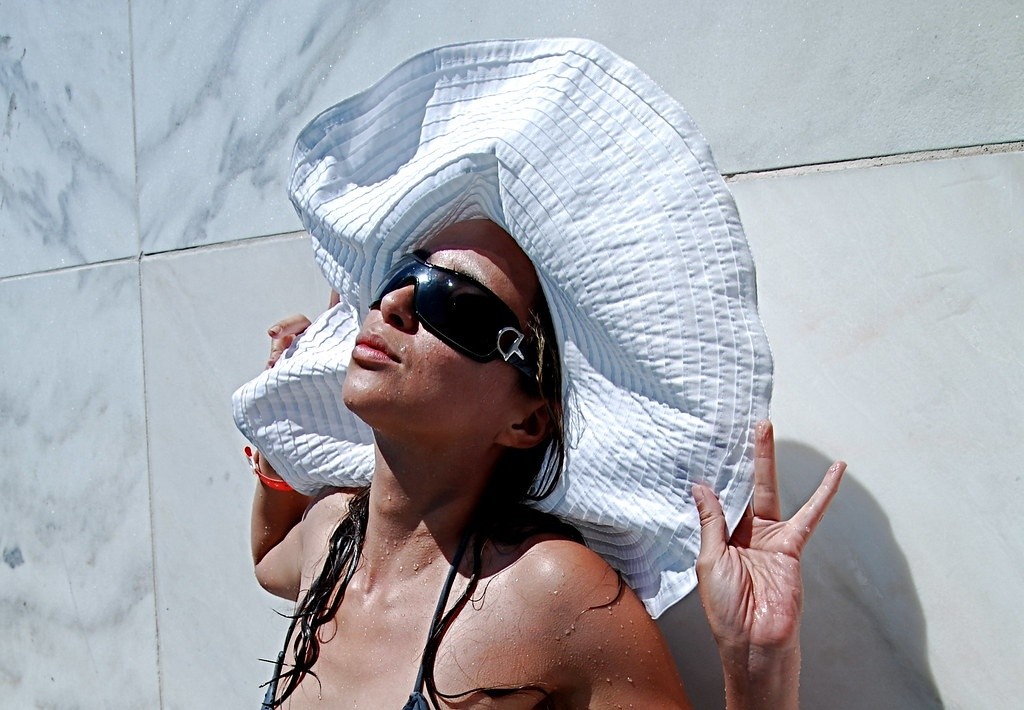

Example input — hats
[224,37,774,620]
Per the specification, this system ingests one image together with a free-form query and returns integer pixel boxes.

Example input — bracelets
[244,447,294,491]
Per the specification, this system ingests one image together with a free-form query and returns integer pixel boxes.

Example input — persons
[232,36,847,710]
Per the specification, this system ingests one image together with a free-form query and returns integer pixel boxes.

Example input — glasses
[366,252,552,396]
